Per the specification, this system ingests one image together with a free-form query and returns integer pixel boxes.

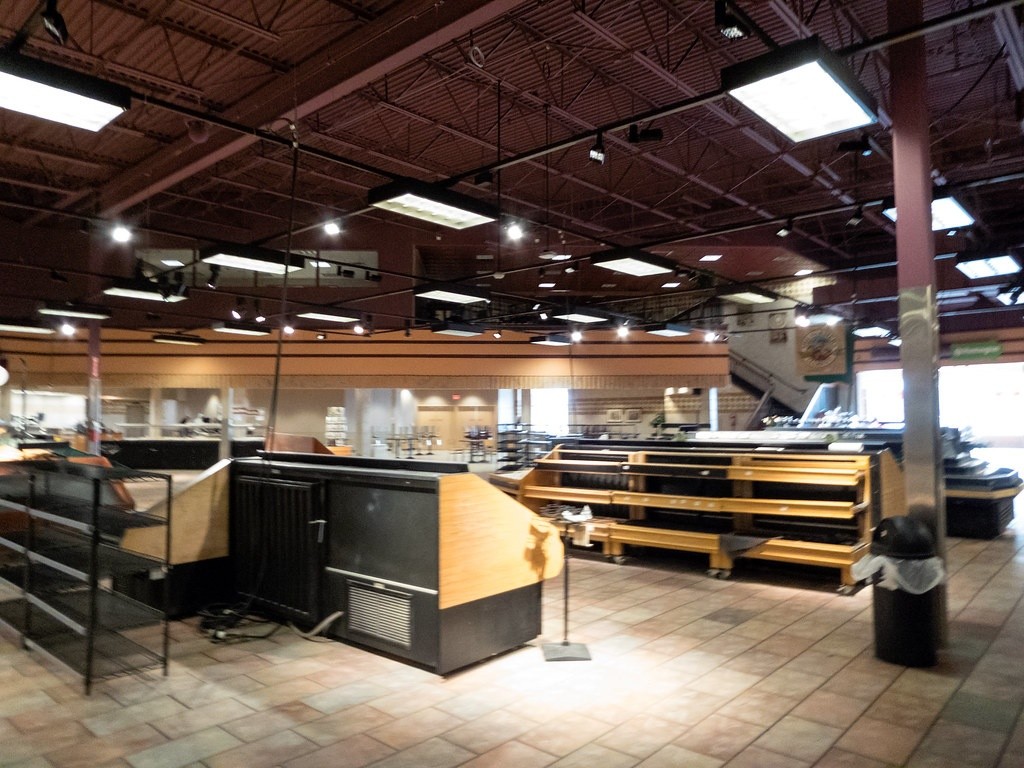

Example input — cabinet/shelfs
[496,430,556,474]
[0,458,173,697]
[558,438,908,521]
[230,444,562,678]
[516,441,879,593]
[65,440,238,621]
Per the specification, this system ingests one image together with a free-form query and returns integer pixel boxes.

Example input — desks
[459,431,492,462]
[385,433,441,458]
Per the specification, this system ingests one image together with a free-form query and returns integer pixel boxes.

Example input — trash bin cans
[868,515,937,668]
